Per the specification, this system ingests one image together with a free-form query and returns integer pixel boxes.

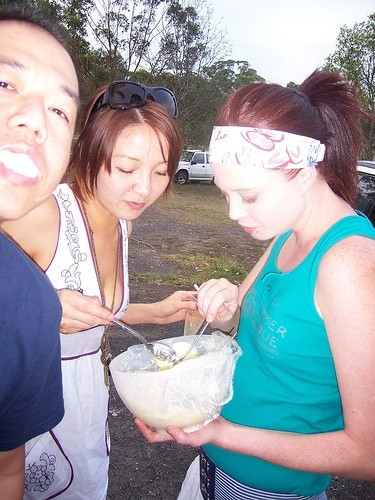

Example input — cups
[183,295,206,337]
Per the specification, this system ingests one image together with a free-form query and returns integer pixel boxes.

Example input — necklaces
[79,198,121,384]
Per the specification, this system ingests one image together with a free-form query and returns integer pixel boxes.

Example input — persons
[0,3,82,499]
[135,67,375,500]
[2,76,200,499]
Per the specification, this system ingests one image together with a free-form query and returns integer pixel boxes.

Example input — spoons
[111,317,177,362]
[172,319,209,366]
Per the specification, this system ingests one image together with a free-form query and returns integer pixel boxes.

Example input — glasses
[89,80,178,121]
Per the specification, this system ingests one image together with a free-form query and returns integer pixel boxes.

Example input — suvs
[174,150,216,186]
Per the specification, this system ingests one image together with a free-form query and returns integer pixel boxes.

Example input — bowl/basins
[109,334,232,435]
